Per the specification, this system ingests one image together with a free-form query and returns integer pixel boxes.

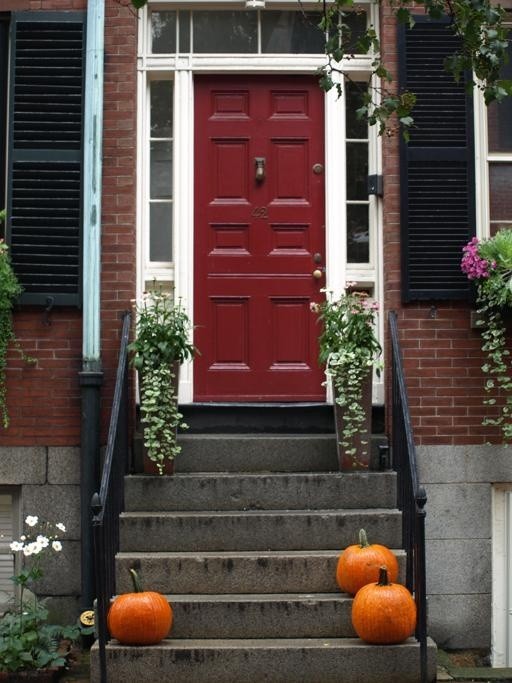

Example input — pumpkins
[336,528,399,596]
[107,567,173,645]
[351,566,418,645]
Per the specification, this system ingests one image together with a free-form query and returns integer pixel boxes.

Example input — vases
[0,639,73,683]
[330,363,375,473]
[136,355,179,478]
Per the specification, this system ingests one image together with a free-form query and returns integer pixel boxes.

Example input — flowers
[308,280,384,469]
[458,228,512,450]
[128,274,206,477]
[0,515,79,672]
